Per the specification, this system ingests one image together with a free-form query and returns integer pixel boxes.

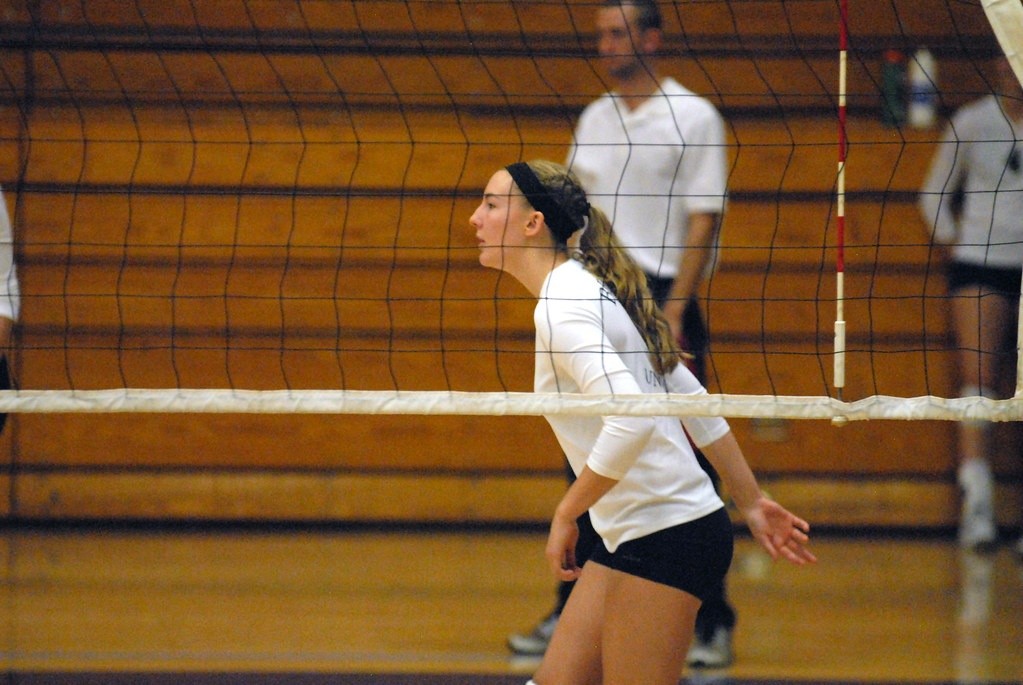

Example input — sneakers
[959,500,996,545]
[506,607,565,655]
[689,625,736,667]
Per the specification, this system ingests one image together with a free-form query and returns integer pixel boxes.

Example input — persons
[1,193,24,435]
[506,0,739,668]
[463,160,809,685]
[914,42,1023,536]
